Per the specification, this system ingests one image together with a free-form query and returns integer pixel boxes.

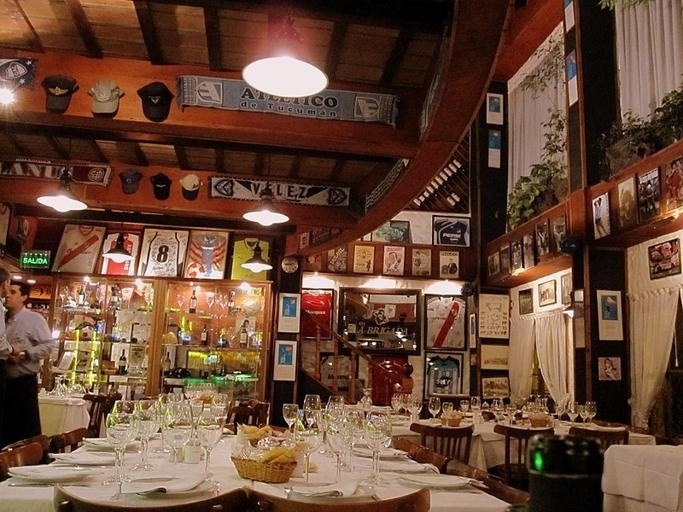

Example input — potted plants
[504,112,564,228]
[599,75,682,177]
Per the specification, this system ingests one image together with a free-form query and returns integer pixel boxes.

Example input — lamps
[238,1,329,100]
[236,147,290,226]
[239,226,273,274]
[34,134,88,215]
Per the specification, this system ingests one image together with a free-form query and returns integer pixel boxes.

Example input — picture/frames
[422,350,464,401]
[319,351,360,392]
[45,219,232,282]
[422,293,467,352]
[300,287,333,341]
[338,287,423,358]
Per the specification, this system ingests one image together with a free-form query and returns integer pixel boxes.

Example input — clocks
[281,253,298,275]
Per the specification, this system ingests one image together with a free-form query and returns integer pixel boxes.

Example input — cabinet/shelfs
[22,270,273,410]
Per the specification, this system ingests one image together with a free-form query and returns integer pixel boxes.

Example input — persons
[386,252,399,273]
[0,279,54,479]
[591,158,682,240]
[0,266,19,361]
[486,216,567,277]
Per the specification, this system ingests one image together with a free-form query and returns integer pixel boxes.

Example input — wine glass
[281,394,393,488]
[103,391,232,500]
[143,287,155,312]
[390,392,598,428]
[51,372,85,400]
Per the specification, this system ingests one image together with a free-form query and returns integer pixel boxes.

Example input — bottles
[53,285,128,376]
[163,283,250,377]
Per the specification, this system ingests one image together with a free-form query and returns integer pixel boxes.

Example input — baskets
[440,417,461,427]
[529,418,549,427]
[230,454,298,484]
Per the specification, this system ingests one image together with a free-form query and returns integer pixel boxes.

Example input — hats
[88,80,124,114]
[136,81,175,122]
[40,75,80,113]
[118,168,143,195]
[150,172,172,200]
[179,174,204,200]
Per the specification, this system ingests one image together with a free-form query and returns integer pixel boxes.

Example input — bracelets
[10,345,14,354]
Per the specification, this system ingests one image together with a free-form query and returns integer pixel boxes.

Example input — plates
[350,444,473,489]
[6,435,135,483]
